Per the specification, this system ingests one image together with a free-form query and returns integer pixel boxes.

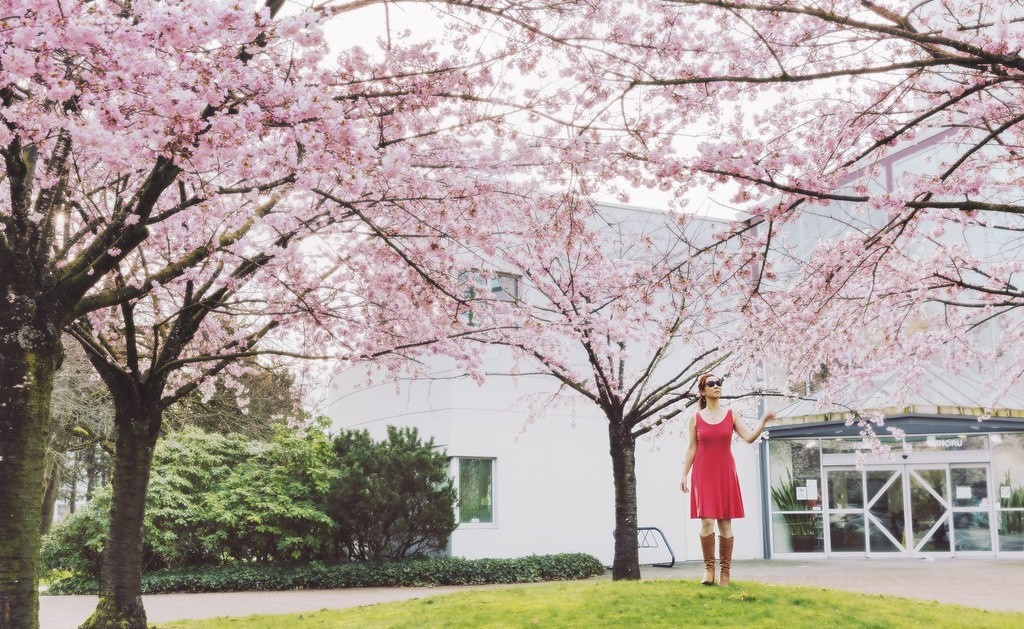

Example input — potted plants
[771,467,816,552]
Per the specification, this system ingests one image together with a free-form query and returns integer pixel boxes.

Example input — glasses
[703,380,723,387]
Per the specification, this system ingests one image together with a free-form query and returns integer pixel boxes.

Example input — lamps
[901,451,908,460]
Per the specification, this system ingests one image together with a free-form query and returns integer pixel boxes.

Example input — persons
[680,373,776,588]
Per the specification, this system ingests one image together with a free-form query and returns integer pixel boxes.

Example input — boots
[699,532,715,584]
[718,535,733,586]
